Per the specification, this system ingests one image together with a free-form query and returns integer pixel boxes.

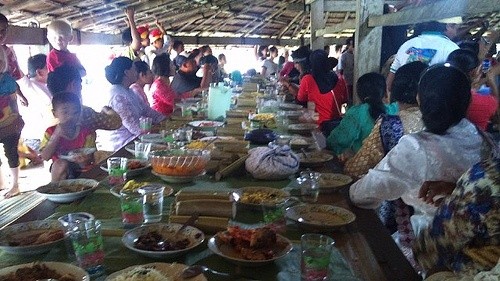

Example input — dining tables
[0,75,424,281]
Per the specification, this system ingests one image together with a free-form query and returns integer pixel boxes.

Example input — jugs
[208,82,230,122]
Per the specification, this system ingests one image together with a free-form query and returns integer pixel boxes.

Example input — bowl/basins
[148,149,210,176]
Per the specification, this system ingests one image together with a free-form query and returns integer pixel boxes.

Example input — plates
[0,82,355,281]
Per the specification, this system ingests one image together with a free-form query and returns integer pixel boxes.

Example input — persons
[39,93,98,184]
[412,111,500,281]
[104,57,164,153]
[349,62,485,271]
[48,64,123,165]
[16,53,58,163]
[0,11,28,198]
[45,20,87,79]
[117,6,500,158]
[345,60,428,232]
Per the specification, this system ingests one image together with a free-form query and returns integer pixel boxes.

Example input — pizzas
[214,225,279,260]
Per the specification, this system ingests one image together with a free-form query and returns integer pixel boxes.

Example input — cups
[69,220,106,277]
[299,171,321,203]
[276,94,286,102]
[141,185,165,224]
[275,118,290,133]
[161,129,177,143]
[134,140,151,160]
[274,73,281,80]
[121,190,145,227]
[139,116,153,133]
[181,105,193,117]
[107,157,127,175]
[178,126,193,143]
[276,135,292,151]
[300,234,335,281]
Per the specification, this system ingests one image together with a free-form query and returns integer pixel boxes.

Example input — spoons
[182,264,247,279]
[153,213,200,251]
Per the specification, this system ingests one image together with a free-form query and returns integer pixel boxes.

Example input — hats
[149,28,163,43]
[137,26,149,39]
[175,48,199,67]
